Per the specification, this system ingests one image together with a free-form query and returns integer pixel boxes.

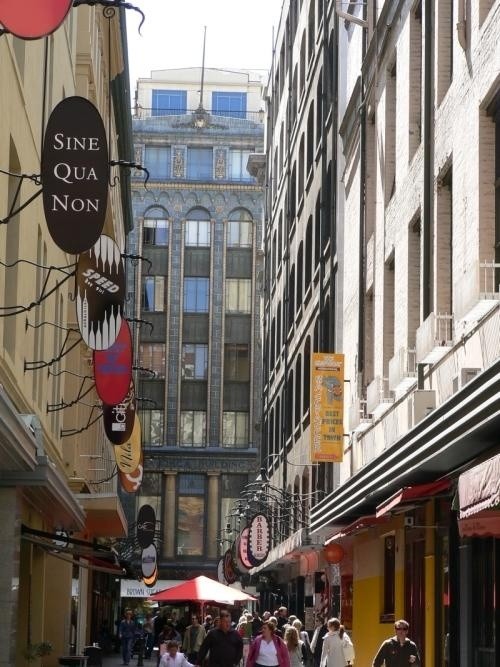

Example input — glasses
[395,627,408,630]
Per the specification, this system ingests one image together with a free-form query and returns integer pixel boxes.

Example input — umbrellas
[147,575,258,622]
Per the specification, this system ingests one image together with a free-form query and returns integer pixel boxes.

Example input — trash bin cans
[58,646,104,667]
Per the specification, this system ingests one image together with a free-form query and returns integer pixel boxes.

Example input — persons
[372,619,421,667]
[119,606,355,667]
